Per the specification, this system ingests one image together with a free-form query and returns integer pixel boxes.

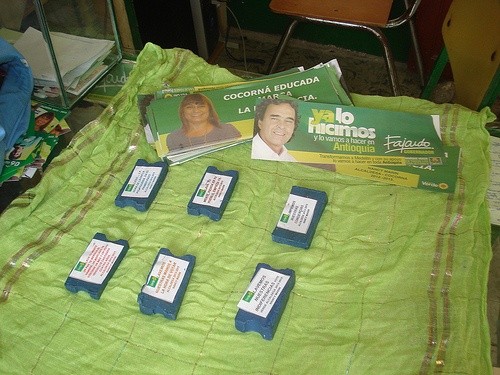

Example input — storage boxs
[0,0,123,112]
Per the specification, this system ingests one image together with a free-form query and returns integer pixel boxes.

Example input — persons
[8,112,70,181]
[251,99,300,162]
[167,94,243,152]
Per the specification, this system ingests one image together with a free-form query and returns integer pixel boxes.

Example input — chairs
[267,0,426,97]
[419,0,500,112]
